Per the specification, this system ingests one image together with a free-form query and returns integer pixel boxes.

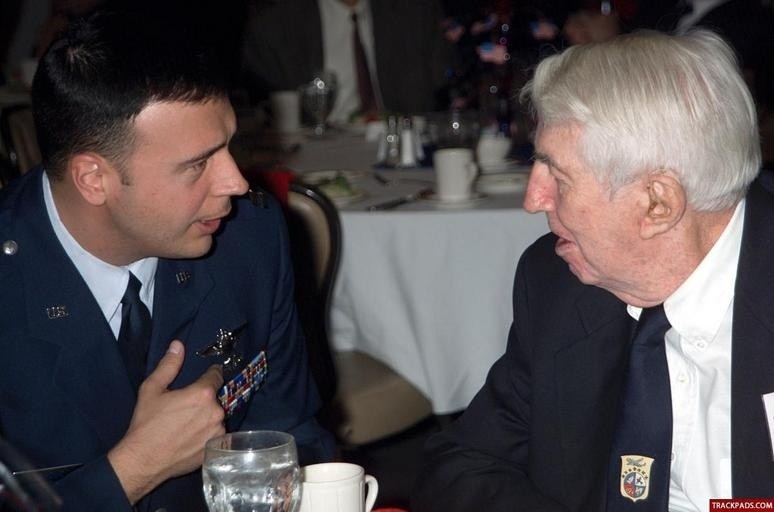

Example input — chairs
[1,100,42,178]
[232,175,344,314]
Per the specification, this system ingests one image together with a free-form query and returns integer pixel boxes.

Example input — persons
[401,26,773,511]
[0,1,336,512]
[252,2,436,126]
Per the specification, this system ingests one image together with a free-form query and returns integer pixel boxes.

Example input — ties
[605,302,673,512]
[119,270,153,392]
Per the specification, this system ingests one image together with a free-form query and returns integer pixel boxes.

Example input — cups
[431,148,478,203]
[298,462,380,512]
[199,429,301,511]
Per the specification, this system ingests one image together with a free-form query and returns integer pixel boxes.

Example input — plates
[418,192,490,209]
[331,193,367,208]
